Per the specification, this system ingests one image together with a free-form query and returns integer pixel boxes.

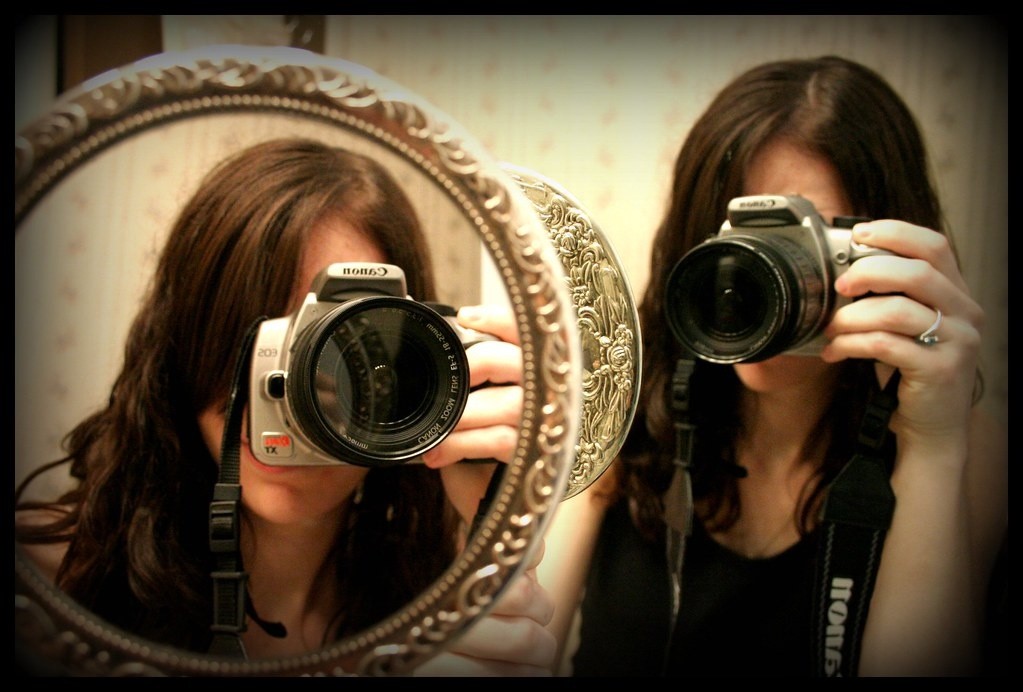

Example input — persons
[410,53,1009,679]
[19,139,526,665]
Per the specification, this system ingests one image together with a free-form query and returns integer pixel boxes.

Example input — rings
[917,308,941,344]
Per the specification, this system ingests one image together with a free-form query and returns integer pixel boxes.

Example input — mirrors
[12,49,643,676]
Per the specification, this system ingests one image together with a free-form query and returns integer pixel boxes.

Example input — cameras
[247,263,513,466]
[662,194,899,365]
